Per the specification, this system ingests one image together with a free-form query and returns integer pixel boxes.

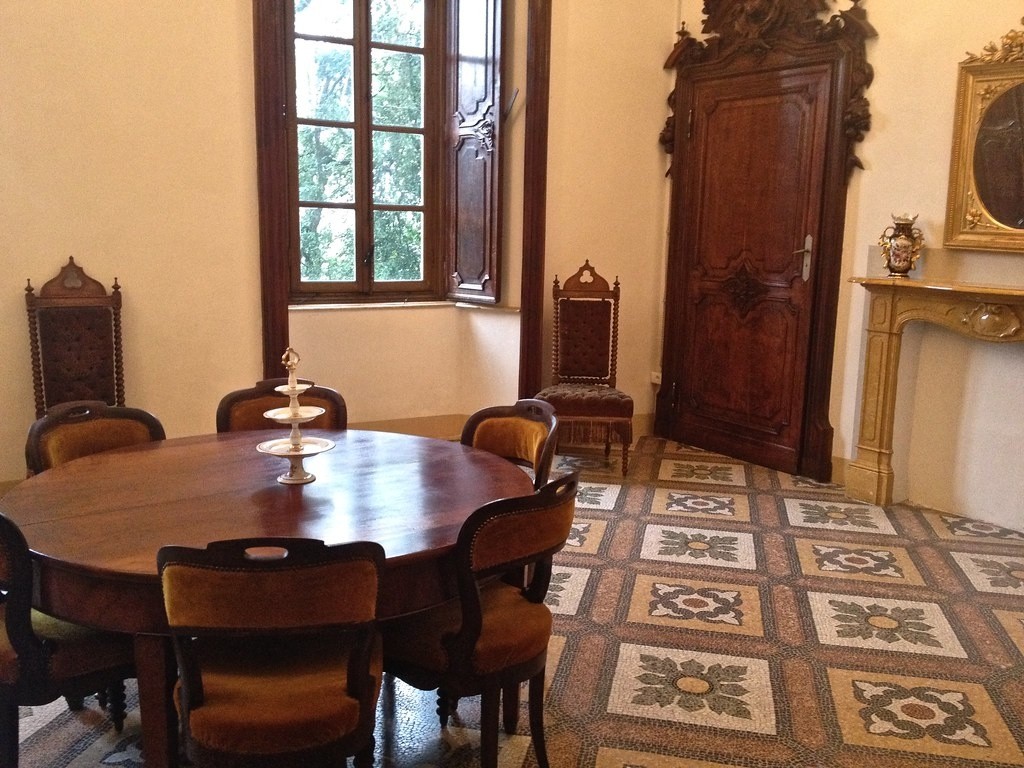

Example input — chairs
[460,399,558,588]
[25,254,126,419]
[23,400,165,477]
[380,471,585,768]
[155,534,387,768]
[0,514,139,768]
[212,376,347,434]
[531,259,638,478]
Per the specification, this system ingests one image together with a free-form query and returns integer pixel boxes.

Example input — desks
[0,431,535,768]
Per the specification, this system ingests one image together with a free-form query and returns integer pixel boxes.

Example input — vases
[878,214,925,278]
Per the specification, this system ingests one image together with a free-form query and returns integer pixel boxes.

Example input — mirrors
[941,28,1024,254]
